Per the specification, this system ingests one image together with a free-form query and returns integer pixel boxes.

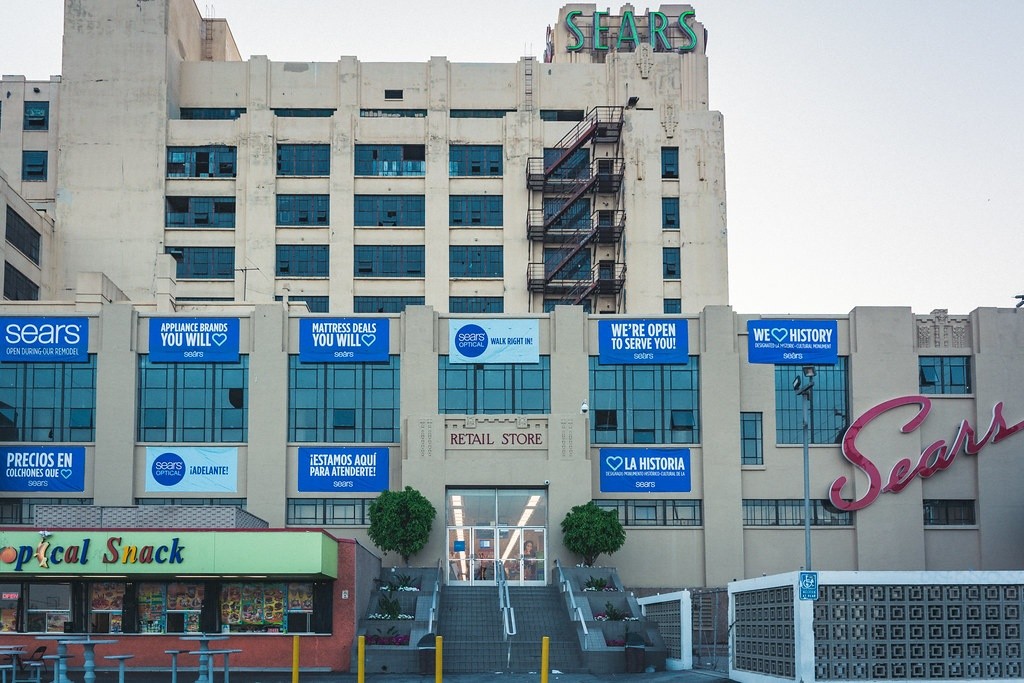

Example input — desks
[0,649,28,683]
[34,635,87,683]
[178,636,231,683]
[58,639,119,683]
[0,643,28,673]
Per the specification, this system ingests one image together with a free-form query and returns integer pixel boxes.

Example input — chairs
[22,646,48,673]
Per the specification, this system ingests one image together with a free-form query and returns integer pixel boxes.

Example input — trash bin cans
[623,643,645,673]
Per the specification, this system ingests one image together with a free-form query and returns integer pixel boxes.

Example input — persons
[508,560,517,580]
[524,540,536,580]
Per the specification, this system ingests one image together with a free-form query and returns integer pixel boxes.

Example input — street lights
[792,364,817,571]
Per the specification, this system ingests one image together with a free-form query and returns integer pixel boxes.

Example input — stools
[42,654,76,683]
[31,661,44,683]
[213,648,244,683]
[0,665,13,683]
[103,653,136,683]
[188,650,231,683]
[165,649,190,683]
[23,661,41,679]
